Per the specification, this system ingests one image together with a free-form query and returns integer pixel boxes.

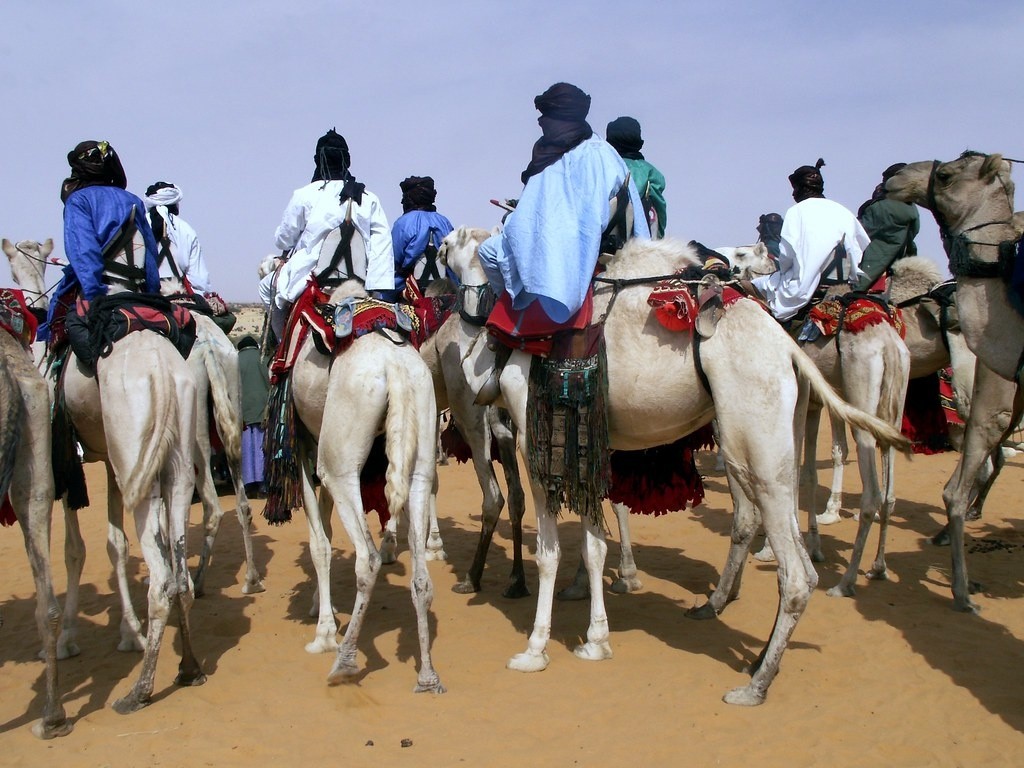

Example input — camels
[1,238,65,741]
[884,150,1024,616]
[710,241,909,599]
[378,278,531,600]
[437,224,917,707]
[555,417,780,621]
[816,255,1024,547]
[256,254,446,695]
[142,277,267,599]
[38,281,214,715]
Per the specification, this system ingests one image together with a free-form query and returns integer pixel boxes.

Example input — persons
[376,173,464,312]
[755,211,785,273]
[255,126,396,386]
[235,335,274,501]
[737,156,872,332]
[476,81,655,355]
[604,116,668,249]
[139,181,213,307]
[41,139,163,386]
[850,162,921,293]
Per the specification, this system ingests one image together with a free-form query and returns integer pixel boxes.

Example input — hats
[881,161,907,177]
[399,175,437,211]
[604,116,645,151]
[788,158,825,196]
[67,140,126,188]
[310,128,356,183]
[534,82,592,122]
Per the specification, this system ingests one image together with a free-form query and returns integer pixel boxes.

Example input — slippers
[695,274,724,338]
